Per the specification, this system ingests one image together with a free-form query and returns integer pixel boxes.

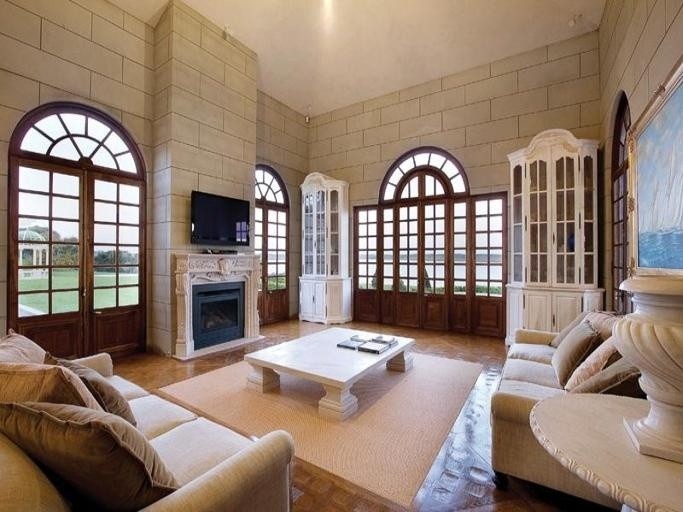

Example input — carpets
[156,350,484,509]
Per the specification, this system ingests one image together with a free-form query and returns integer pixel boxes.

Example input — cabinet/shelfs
[297,171,354,326]
[503,128,606,347]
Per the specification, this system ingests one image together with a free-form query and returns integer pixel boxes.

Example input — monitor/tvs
[190,189,251,246]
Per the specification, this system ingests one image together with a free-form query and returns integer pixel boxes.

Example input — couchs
[0,351,295,511]
[490,310,646,510]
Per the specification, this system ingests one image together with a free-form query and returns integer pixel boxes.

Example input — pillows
[0,328,180,512]
[549,319,646,399]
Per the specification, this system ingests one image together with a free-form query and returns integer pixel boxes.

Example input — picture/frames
[625,54,683,279]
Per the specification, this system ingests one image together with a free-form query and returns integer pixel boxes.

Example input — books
[336,334,398,354]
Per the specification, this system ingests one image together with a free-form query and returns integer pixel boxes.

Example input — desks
[528,392,683,512]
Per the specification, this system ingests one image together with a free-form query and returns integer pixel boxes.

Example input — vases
[611,273,683,464]
